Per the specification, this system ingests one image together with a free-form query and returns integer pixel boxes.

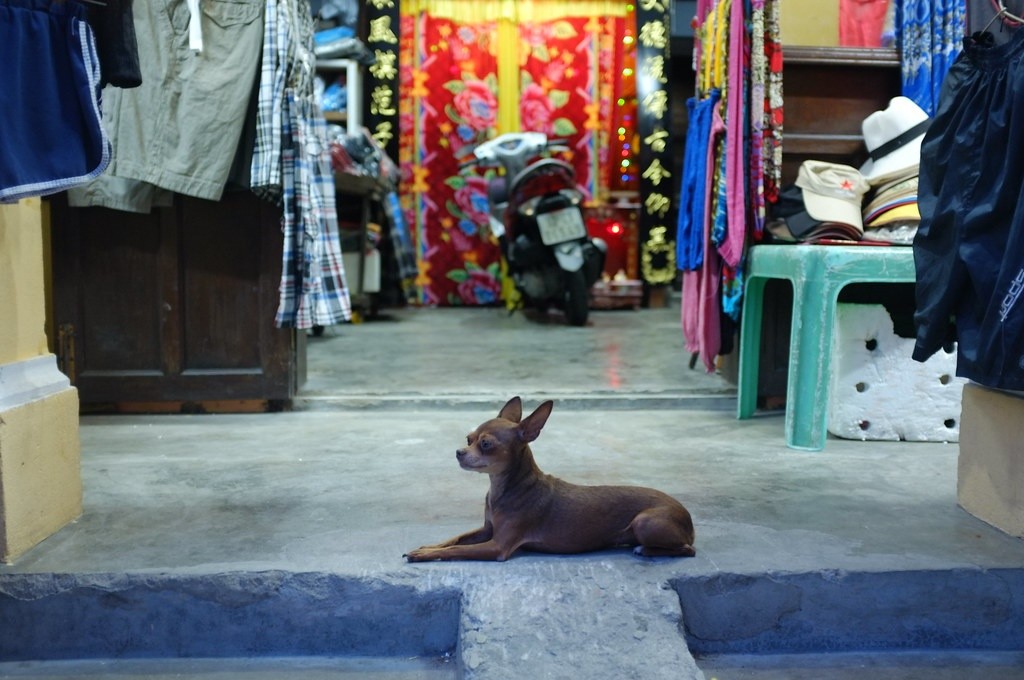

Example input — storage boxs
[828,302,971,442]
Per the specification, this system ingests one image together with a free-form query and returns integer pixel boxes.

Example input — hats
[764,159,871,242]
[861,96,932,245]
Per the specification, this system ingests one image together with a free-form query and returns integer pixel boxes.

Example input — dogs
[399,397,696,563]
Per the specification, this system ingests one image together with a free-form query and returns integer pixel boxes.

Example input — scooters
[455,129,609,327]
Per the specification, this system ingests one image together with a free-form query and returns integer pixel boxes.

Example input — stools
[735,243,916,450]
